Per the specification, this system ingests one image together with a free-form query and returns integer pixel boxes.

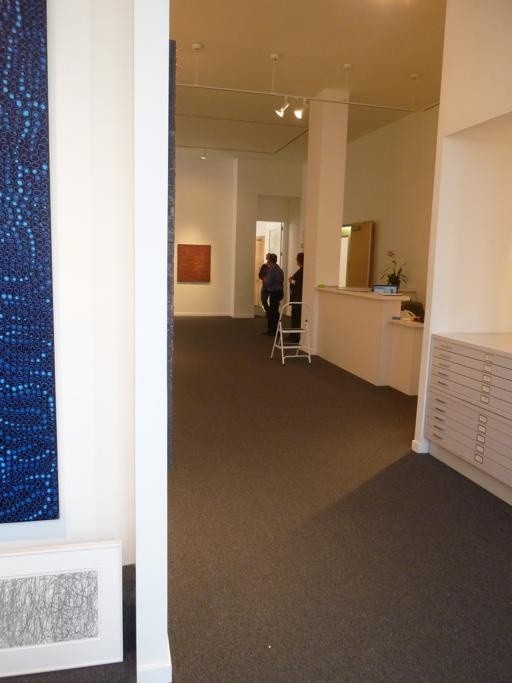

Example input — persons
[258,252,271,317]
[260,252,284,336]
[284,251,305,341]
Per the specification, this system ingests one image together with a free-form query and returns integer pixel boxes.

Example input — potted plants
[379,249,409,292]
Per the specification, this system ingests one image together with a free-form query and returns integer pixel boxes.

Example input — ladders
[270,302,312,366]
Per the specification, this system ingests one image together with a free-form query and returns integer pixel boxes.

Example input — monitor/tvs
[371,284,398,293]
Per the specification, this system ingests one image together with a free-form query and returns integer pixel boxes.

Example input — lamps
[274,95,310,119]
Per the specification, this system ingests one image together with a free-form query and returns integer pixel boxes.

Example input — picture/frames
[0,537,125,679]
